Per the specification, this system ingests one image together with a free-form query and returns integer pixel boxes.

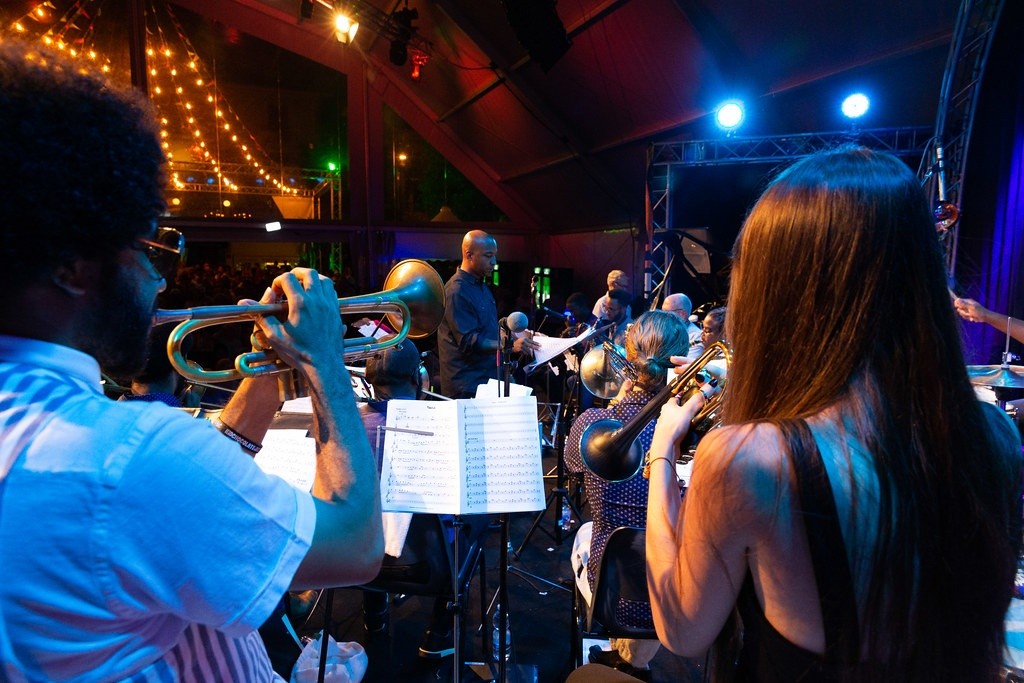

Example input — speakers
[438,1,572,86]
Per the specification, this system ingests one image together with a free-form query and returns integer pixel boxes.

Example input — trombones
[579,336,640,400]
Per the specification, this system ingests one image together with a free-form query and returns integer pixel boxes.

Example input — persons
[661,293,705,387]
[593,270,631,320]
[563,291,604,348]
[601,289,633,343]
[354,334,424,477]
[701,308,727,350]
[118,325,184,411]
[0,32,384,683]
[565,311,697,675]
[156,228,356,308]
[644,144,1023,683]
[439,230,541,399]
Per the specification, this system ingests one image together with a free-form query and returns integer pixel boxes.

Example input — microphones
[935,141,948,203]
[499,311,528,334]
[543,305,575,323]
[420,349,432,358]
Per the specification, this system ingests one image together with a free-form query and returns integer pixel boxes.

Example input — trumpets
[146,258,446,387]
[575,335,733,484]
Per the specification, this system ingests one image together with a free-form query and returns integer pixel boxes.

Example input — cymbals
[964,365,1023,390]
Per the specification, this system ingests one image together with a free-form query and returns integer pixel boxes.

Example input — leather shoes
[285,588,317,629]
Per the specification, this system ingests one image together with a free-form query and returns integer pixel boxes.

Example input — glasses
[661,308,683,313]
[601,303,619,314]
[698,322,720,336]
[614,281,628,289]
[113,215,188,283]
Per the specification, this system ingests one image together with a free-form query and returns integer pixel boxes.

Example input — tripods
[510,363,588,562]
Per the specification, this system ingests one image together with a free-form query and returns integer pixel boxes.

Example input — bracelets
[642,452,685,487]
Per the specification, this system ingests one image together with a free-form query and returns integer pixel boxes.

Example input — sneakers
[362,592,389,635]
[416,626,461,661]
